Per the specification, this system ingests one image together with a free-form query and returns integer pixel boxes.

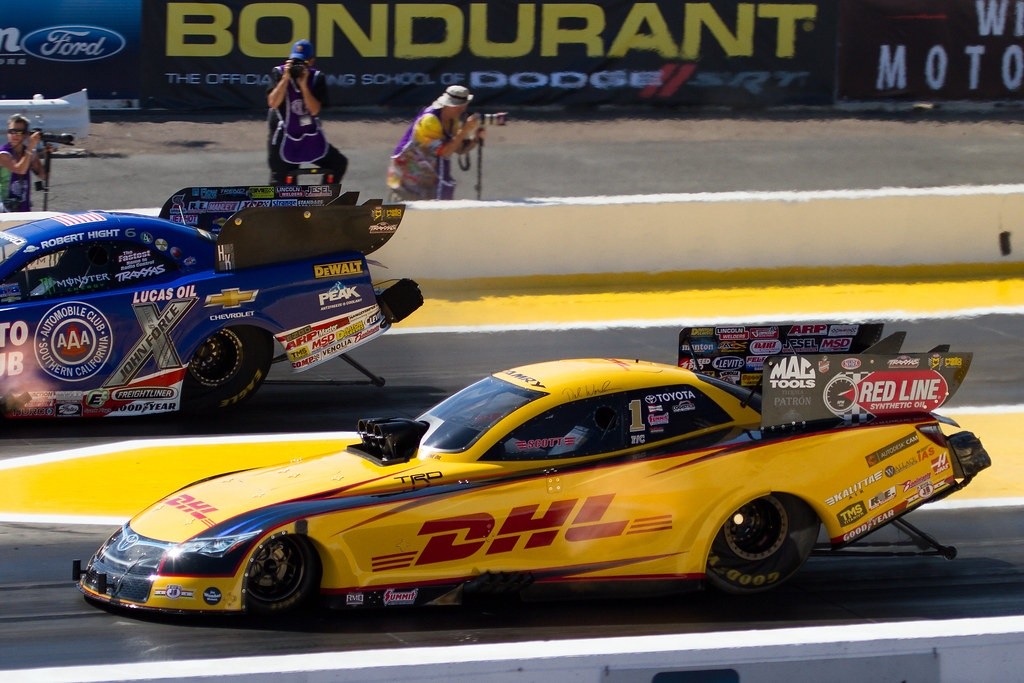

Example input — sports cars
[70,322,994,621]
[1,185,423,421]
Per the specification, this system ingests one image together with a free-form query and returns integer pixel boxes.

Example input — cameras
[27,127,74,147]
[287,57,305,78]
[462,110,510,130]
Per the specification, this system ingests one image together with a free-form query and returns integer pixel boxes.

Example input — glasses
[7,128,26,134]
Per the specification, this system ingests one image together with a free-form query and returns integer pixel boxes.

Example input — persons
[266,39,349,187]
[1,113,55,215]
[387,86,481,200]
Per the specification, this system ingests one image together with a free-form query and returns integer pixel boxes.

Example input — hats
[289,39,314,59]
[437,85,473,106]
